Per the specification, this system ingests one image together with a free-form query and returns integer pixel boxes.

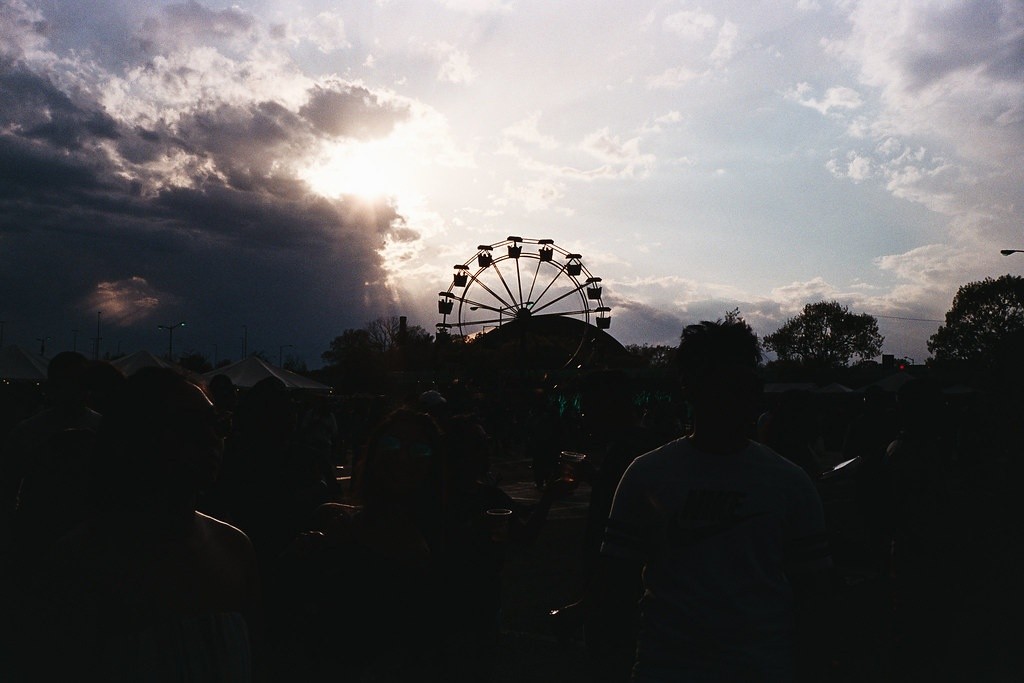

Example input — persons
[0,316,1022,683]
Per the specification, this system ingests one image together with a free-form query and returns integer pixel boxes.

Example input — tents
[108,350,176,378]
[199,352,333,393]
[0,343,51,381]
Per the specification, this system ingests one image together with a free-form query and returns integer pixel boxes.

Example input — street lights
[279,344,293,367]
[470,302,533,350]
[36,336,50,358]
[157,322,189,367]
